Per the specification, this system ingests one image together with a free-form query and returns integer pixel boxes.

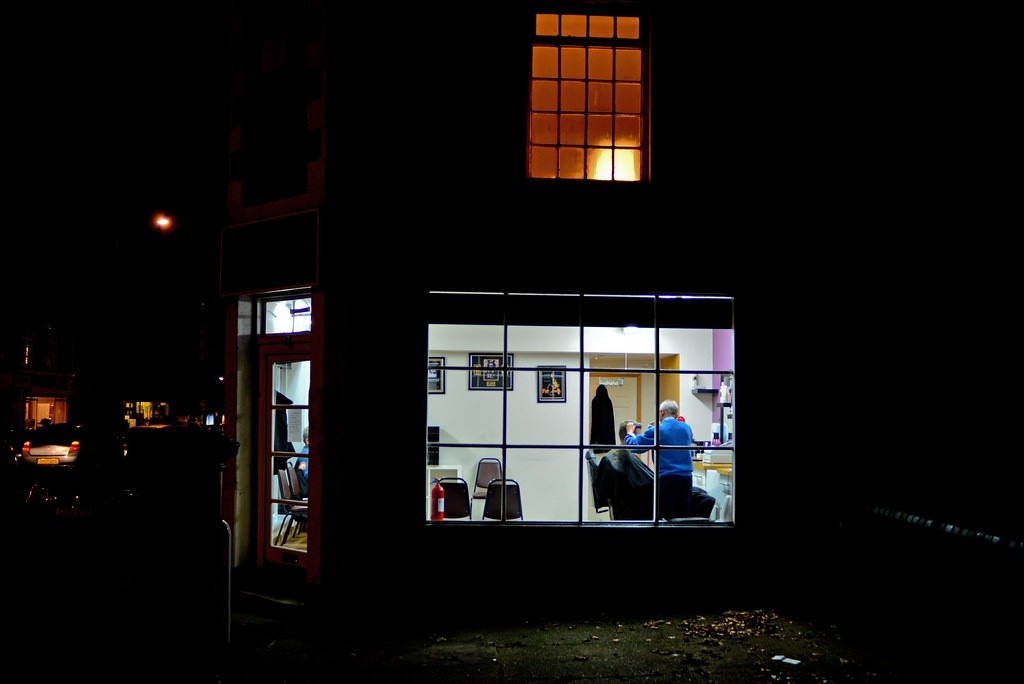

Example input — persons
[294,429,309,527]
[624,399,694,520]
[607,420,715,523]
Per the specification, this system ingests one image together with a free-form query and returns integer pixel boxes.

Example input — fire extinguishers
[430,477,445,520]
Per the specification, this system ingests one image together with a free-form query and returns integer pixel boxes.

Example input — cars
[1,412,223,533]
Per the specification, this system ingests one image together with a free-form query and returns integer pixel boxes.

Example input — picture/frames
[467,353,514,392]
[427,356,446,395]
[536,365,566,403]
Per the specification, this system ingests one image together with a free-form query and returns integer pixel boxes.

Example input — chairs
[584,450,613,519]
[470,458,503,508]
[436,476,473,521]
[481,479,525,521]
[274,461,308,547]
[599,452,654,519]
